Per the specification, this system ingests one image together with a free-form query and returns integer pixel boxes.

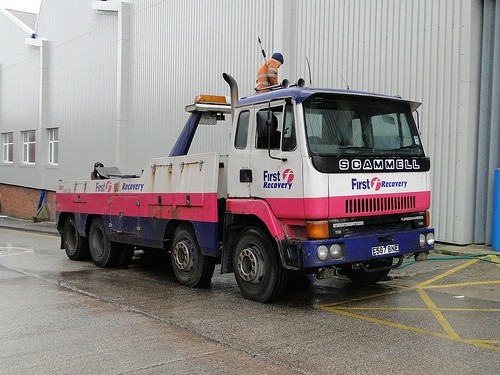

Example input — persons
[256,53,284,92]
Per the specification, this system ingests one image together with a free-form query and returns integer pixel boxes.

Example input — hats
[271,53,284,64]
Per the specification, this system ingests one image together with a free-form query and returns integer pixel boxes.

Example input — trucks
[53,73,436,303]
